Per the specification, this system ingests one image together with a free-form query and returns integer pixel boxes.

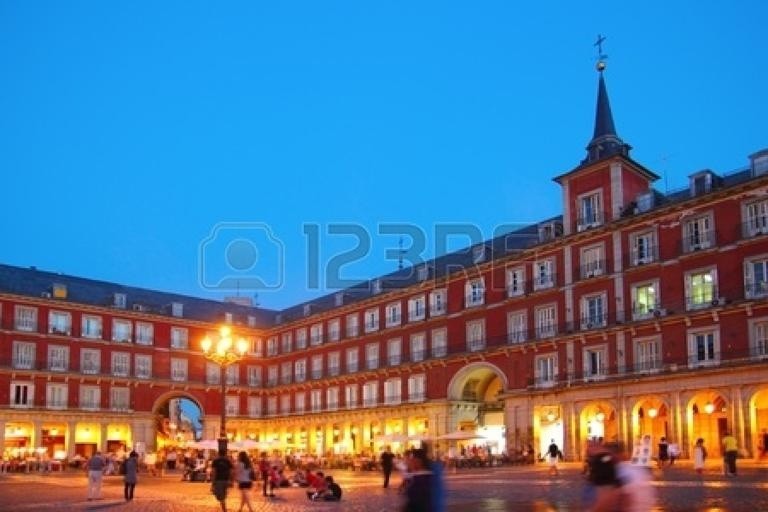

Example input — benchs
[189,472,206,481]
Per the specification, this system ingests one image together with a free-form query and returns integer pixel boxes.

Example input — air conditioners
[709,297,726,307]
[581,323,595,330]
[133,304,142,311]
[650,308,667,318]
[689,244,704,252]
[41,291,50,298]
[634,257,652,265]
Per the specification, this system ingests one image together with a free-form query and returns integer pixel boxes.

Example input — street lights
[199,325,248,457]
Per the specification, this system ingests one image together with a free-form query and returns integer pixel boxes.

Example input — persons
[690,436,709,476]
[718,430,739,476]
[720,447,732,474]
